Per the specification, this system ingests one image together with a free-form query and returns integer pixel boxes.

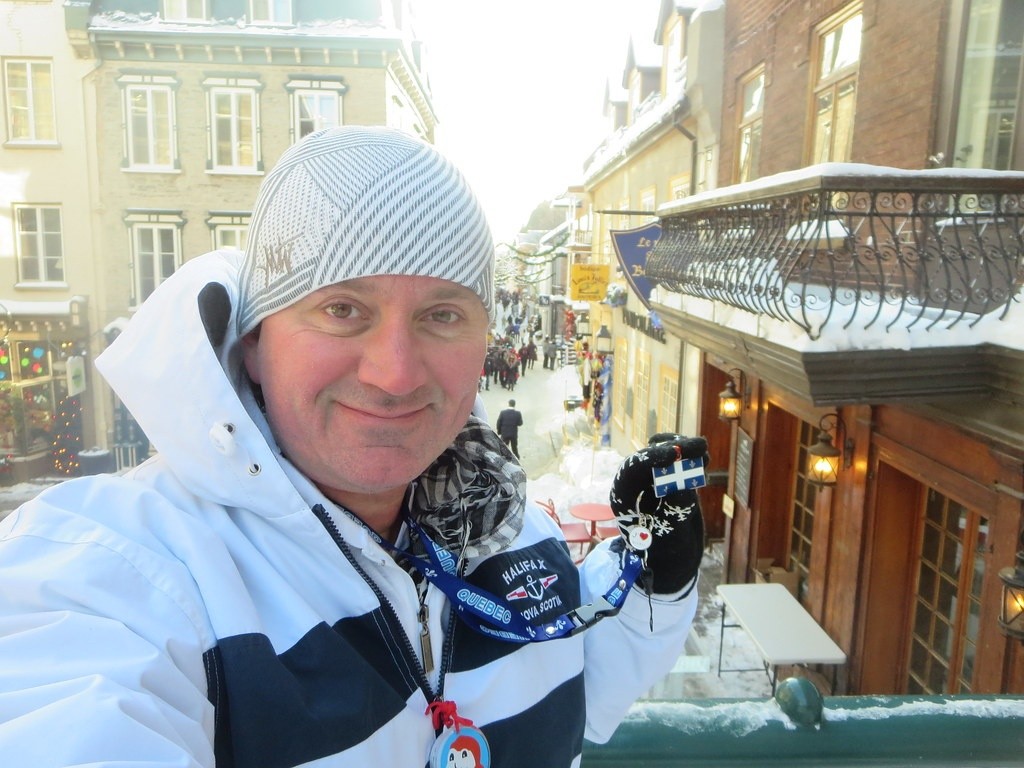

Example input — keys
[643,567,663,631]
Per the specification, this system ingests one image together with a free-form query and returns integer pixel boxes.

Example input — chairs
[595,524,622,541]
[535,499,592,554]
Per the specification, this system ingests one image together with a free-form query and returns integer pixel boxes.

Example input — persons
[543,334,558,370]
[494,399,522,460]
[0,123,712,768]
[481,285,541,390]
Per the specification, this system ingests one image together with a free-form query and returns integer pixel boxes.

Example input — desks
[569,503,617,564]
[716,583,848,699]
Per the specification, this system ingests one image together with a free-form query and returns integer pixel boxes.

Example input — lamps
[595,325,614,355]
[997,527,1024,642]
[577,312,592,336]
[806,413,854,492]
[718,368,751,428]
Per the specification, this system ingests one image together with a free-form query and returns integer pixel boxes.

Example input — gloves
[610,433,709,595]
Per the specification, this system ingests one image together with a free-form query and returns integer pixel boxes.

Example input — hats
[233,125,495,340]
